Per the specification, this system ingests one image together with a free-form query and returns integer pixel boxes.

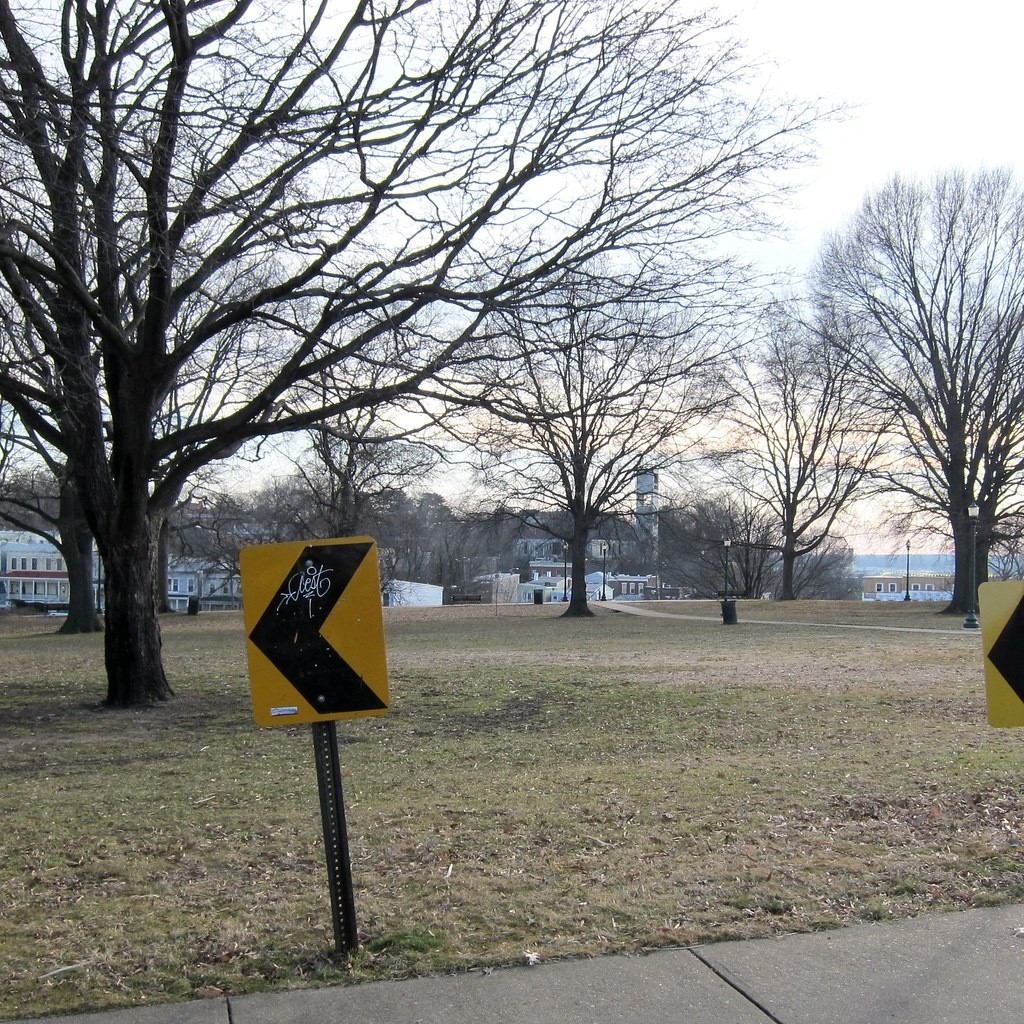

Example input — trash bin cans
[188,596,199,615]
[533,588,544,604]
[719,597,738,625]
